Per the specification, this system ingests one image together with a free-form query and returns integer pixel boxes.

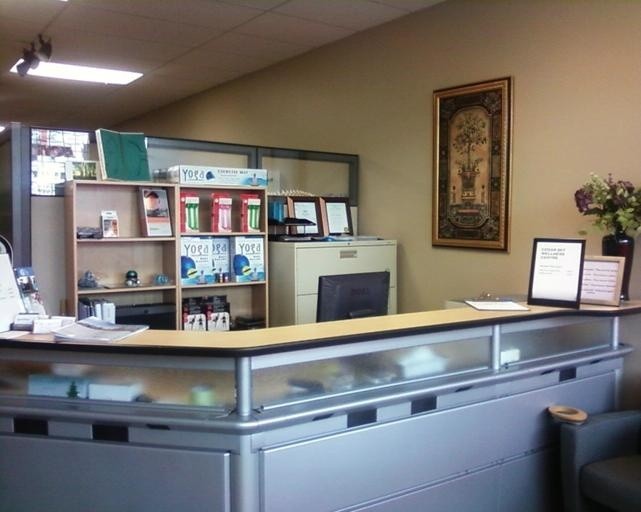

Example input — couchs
[561,410,641,511]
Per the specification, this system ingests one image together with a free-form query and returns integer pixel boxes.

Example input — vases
[603,225,635,301]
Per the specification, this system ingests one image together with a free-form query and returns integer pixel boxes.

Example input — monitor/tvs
[316,271,390,324]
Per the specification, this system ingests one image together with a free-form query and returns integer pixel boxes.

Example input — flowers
[571,172,641,238]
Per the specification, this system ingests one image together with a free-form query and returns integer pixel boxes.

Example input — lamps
[16,34,53,78]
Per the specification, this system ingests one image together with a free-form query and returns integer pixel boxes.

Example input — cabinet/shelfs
[179,184,271,331]
[65,179,179,329]
[268,240,398,326]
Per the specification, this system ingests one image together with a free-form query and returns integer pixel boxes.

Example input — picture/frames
[430,74,514,253]
[527,238,587,310]
[319,197,353,237]
[581,255,626,306]
[287,195,324,237]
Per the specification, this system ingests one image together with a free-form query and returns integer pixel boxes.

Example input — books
[51,315,149,345]
